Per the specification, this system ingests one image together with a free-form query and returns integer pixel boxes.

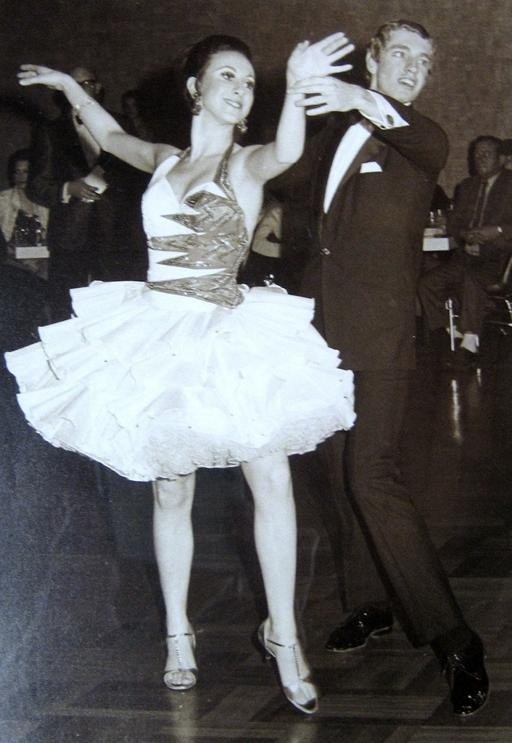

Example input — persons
[284,18,492,718]
[3,30,358,714]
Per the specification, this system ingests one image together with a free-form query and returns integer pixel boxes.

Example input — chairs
[444,247,511,353]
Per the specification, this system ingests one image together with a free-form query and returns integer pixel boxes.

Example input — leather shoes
[324,603,393,653]
[446,632,491,718]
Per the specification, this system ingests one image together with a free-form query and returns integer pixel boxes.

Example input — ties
[473,182,489,230]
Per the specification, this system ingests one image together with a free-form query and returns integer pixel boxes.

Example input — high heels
[257,616,318,714]
[163,622,198,691]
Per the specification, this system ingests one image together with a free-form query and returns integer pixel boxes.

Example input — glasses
[79,79,95,89]
